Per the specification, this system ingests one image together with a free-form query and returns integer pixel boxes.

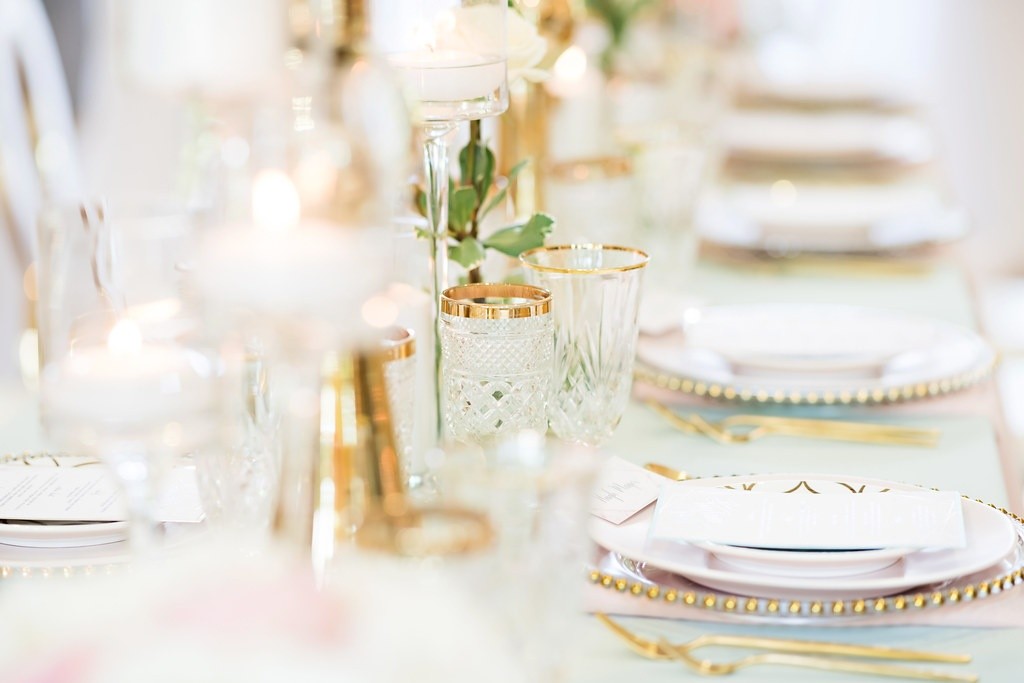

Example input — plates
[0,452,126,549]
[698,80,966,257]
[586,472,1024,618]
[585,473,1018,587]
[677,528,922,581]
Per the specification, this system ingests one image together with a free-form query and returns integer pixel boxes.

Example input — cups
[439,280,555,455]
[518,245,650,452]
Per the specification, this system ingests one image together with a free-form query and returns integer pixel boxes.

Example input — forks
[641,399,936,454]
[595,611,974,664]
[655,637,979,683]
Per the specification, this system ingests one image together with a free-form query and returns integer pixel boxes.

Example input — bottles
[634,333,993,405]
